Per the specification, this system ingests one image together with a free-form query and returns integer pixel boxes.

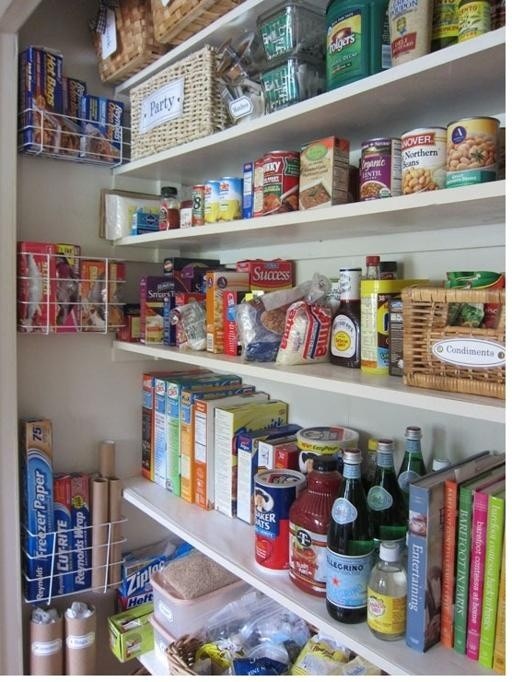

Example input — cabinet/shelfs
[7,103,133,611]
[101,0,506,677]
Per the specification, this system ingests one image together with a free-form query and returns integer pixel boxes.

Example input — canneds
[192,136,358,226]
[296,426,360,476]
[253,467,305,571]
[359,137,401,201]
[401,126,448,195]
[445,117,505,189]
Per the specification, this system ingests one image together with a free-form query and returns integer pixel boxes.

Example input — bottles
[365,426,451,552]
[328,254,398,367]
[326,448,375,625]
[366,542,408,639]
[159,186,181,232]
[288,457,342,602]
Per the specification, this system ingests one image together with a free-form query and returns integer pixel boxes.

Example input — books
[405,450,505,675]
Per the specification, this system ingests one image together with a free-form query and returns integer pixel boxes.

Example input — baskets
[151,1,244,46]
[402,285,505,401]
[94,0,165,82]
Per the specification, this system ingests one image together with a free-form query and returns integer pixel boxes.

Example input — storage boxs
[143,558,256,641]
[98,2,237,87]
[139,371,306,529]
[146,610,180,669]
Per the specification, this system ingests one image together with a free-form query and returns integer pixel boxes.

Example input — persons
[56,253,79,310]
[255,489,269,513]
[301,452,317,476]
